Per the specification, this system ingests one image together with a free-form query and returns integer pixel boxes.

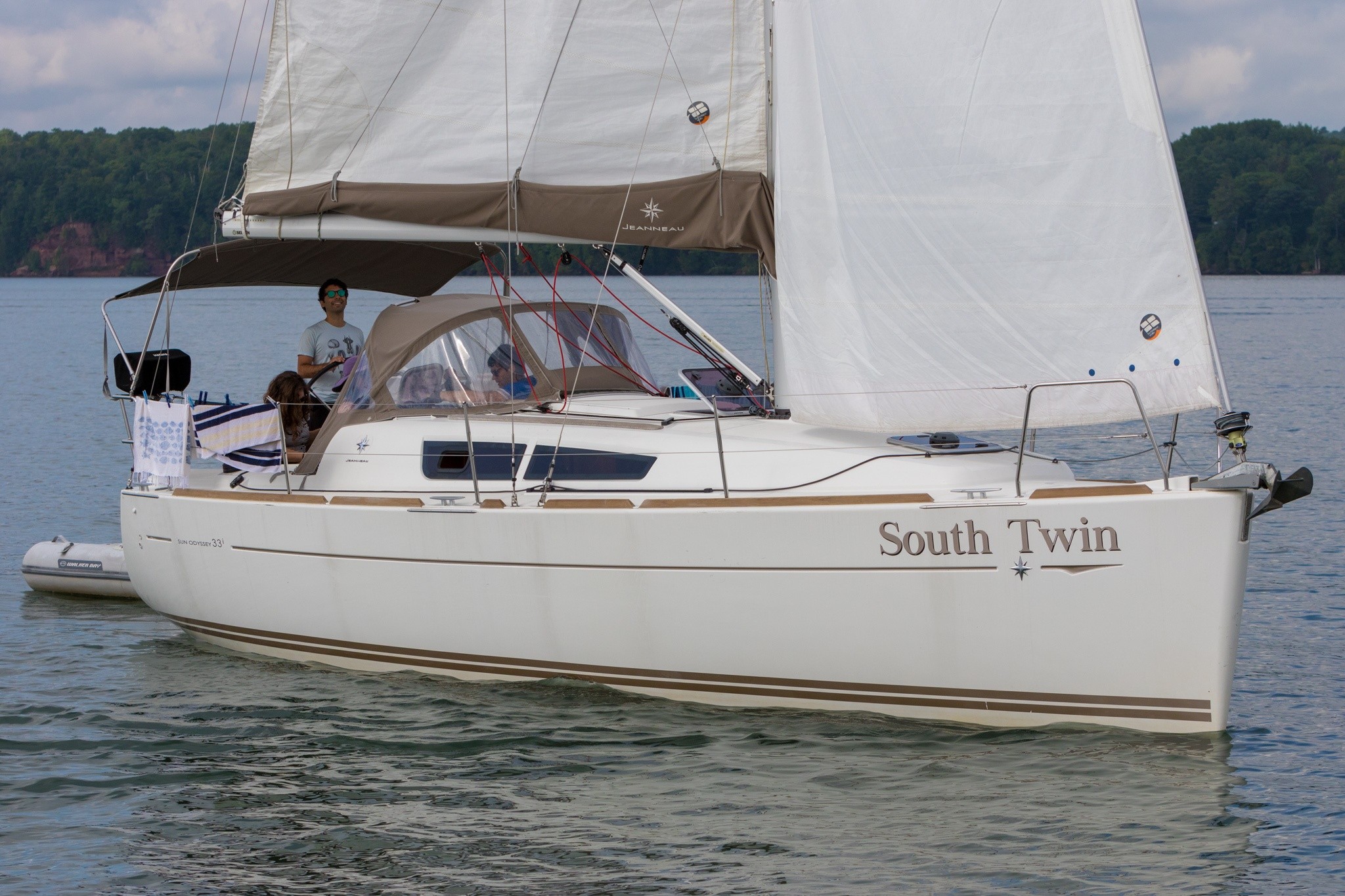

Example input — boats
[19,535,141,599]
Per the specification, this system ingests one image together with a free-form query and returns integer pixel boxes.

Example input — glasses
[299,394,308,403]
[491,367,502,377]
[323,289,346,298]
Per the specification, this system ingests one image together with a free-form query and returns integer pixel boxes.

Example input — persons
[297,277,370,432]
[430,343,537,403]
[263,371,320,464]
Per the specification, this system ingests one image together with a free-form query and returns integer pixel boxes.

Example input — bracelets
[302,453,305,460]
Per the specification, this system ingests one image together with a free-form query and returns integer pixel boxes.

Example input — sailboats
[98,1,1315,733]
